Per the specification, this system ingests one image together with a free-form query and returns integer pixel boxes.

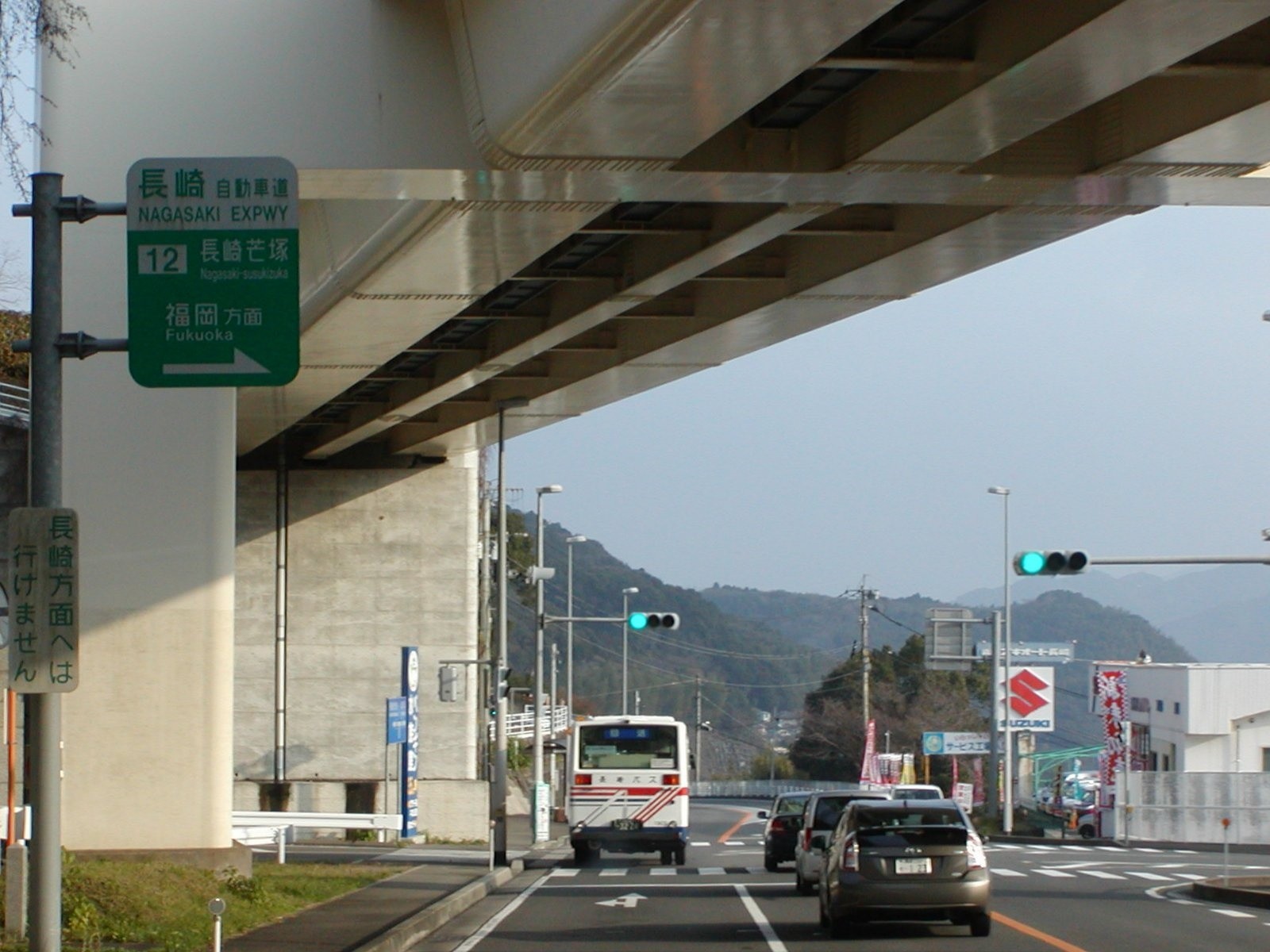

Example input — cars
[889,784,949,826]
[790,789,902,898]
[809,797,990,938]
[1036,769,1102,840]
[757,791,815,873]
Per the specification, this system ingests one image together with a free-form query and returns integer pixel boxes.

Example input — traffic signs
[127,156,300,389]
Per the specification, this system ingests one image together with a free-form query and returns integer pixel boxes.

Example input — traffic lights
[488,694,498,718]
[1012,547,1091,577]
[627,611,681,631]
[492,665,513,703]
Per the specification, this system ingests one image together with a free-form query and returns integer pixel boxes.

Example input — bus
[563,713,697,867]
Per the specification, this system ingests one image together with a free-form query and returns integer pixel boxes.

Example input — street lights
[565,535,587,783]
[988,486,1012,833]
[536,484,563,784]
[622,587,639,715]
